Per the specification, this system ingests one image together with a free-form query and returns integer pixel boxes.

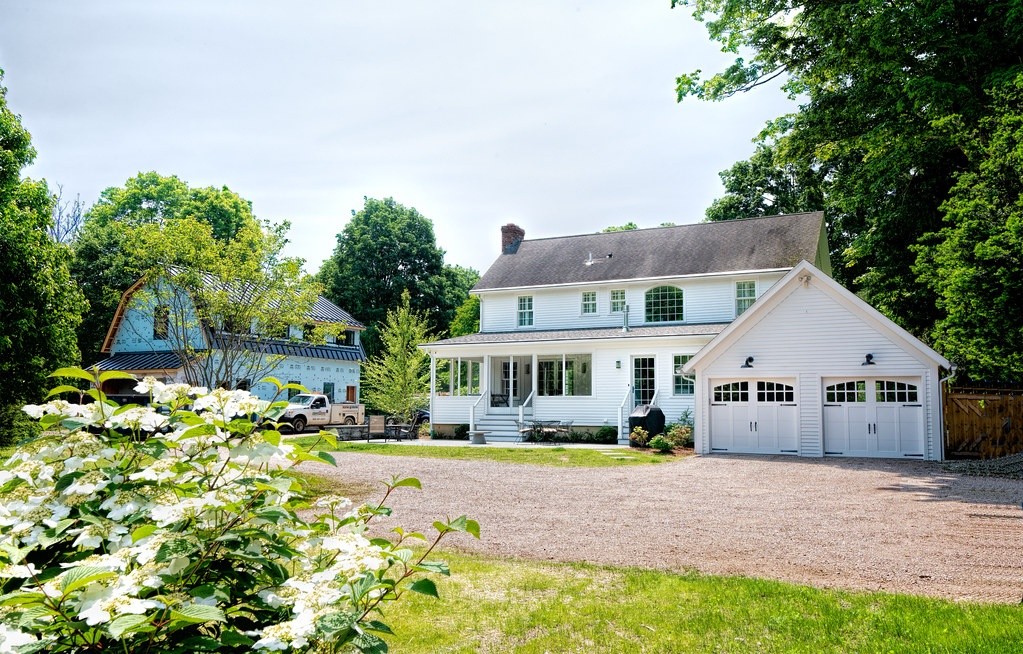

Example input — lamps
[616,360,621,368]
[740,356,754,368]
[860,354,876,366]
[676,366,683,373]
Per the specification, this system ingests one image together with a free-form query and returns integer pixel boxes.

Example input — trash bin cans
[629,404,665,447]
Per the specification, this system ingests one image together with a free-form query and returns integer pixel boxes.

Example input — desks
[324,425,420,442]
[466,431,492,443]
[524,419,561,446]
[468,394,509,407]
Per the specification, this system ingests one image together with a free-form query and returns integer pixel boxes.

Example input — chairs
[399,410,420,441]
[367,414,387,443]
[513,418,574,445]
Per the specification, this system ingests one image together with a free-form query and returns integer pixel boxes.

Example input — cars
[385,407,430,428]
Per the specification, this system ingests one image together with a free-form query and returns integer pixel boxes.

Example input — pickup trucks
[278,393,365,434]
